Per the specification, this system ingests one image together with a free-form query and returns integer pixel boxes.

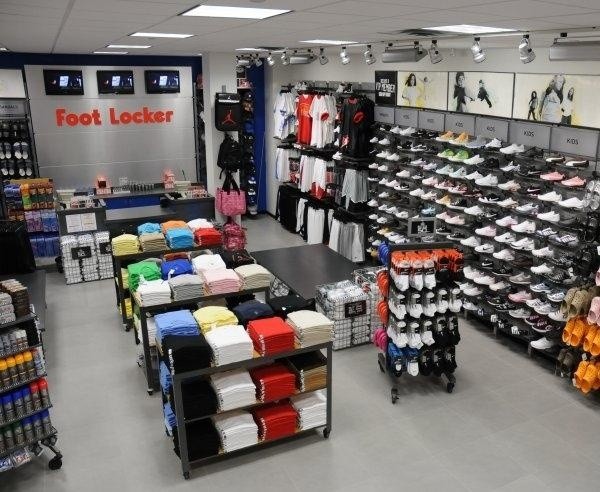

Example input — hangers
[349,89,369,103]
[317,86,335,97]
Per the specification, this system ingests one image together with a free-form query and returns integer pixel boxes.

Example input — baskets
[59,230,115,285]
[352,264,388,344]
[316,278,372,352]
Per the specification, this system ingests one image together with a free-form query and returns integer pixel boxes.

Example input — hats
[242,101,256,112]
[244,147,258,215]
[242,112,254,121]
[242,90,255,101]
[243,135,257,146]
[241,78,250,87]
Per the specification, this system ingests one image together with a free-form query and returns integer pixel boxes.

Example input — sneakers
[462,300,479,312]
[395,237,406,244]
[386,206,396,214]
[474,275,495,286]
[335,114,338,119]
[394,183,410,190]
[412,214,419,218]
[378,178,388,184]
[446,232,465,241]
[508,290,532,303]
[342,135,351,147]
[476,259,493,269]
[474,226,497,236]
[437,148,455,157]
[497,196,519,207]
[410,158,426,166]
[411,129,426,136]
[493,249,516,260]
[389,125,402,133]
[423,162,437,170]
[397,141,413,149]
[379,191,389,198]
[446,199,467,210]
[436,131,454,142]
[411,172,425,179]
[485,137,503,149]
[372,239,380,247]
[449,132,469,145]
[436,193,452,204]
[367,236,374,242]
[558,197,583,207]
[400,127,415,135]
[281,134,296,142]
[378,164,388,171]
[448,149,470,162]
[488,281,511,291]
[464,251,479,261]
[498,179,521,191]
[386,219,399,227]
[525,315,545,325]
[546,292,565,303]
[369,137,379,143]
[369,188,378,196]
[388,193,401,200]
[294,118,300,125]
[368,175,378,181]
[423,236,435,242]
[377,216,387,223]
[366,199,379,207]
[517,184,541,196]
[494,301,516,311]
[332,151,342,160]
[435,212,451,219]
[396,211,408,219]
[389,234,400,242]
[510,237,535,251]
[422,176,438,185]
[511,256,533,267]
[526,298,542,307]
[380,124,391,130]
[370,248,377,257]
[465,170,483,180]
[552,256,575,266]
[494,231,515,244]
[370,121,379,129]
[448,182,467,193]
[495,215,518,227]
[561,175,586,187]
[481,210,497,217]
[464,205,483,216]
[367,223,380,231]
[436,164,453,175]
[537,211,560,223]
[368,163,378,169]
[449,167,466,178]
[515,202,539,215]
[386,152,399,161]
[548,310,570,322]
[545,153,565,164]
[384,231,395,238]
[475,291,491,301]
[423,131,439,138]
[479,193,501,203]
[391,137,401,147]
[445,216,465,225]
[511,219,536,232]
[420,191,437,200]
[518,165,542,179]
[532,246,554,260]
[519,146,543,158]
[463,287,483,296]
[421,206,435,216]
[509,308,532,318]
[478,158,499,169]
[460,282,474,291]
[533,303,559,315]
[376,151,387,158]
[463,220,482,232]
[564,158,589,168]
[509,272,532,284]
[422,146,438,155]
[559,217,580,229]
[475,173,498,187]
[295,82,300,89]
[399,197,409,204]
[378,204,387,210]
[333,125,340,133]
[556,235,579,248]
[531,336,554,350]
[499,160,521,172]
[492,265,513,276]
[460,236,481,247]
[368,213,379,220]
[377,229,386,235]
[343,84,352,93]
[487,296,502,306]
[531,322,553,333]
[536,228,559,238]
[293,96,300,103]
[474,243,494,253]
[385,179,400,186]
[463,189,483,197]
[436,226,451,235]
[463,265,485,280]
[396,170,410,177]
[379,138,390,145]
[411,142,427,152]
[537,191,562,202]
[334,138,339,146]
[368,148,378,157]
[299,81,307,90]
[550,272,573,285]
[465,135,486,148]
[367,247,371,252]
[529,282,553,294]
[336,83,345,93]
[499,143,525,154]
[410,187,425,197]
[530,263,554,278]
[540,171,564,181]
[463,153,484,165]
[434,178,455,189]
[398,157,411,163]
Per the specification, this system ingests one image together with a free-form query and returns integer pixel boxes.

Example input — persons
[453,71,474,114]
[477,80,492,108]
[562,87,575,124]
[540,73,565,124]
[402,73,421,106]
[418,75,434,105]
[527,90,537,120]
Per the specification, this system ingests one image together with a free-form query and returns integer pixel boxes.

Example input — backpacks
[216,93,241,131]
[224,221,247,251]
[218,135,241,172]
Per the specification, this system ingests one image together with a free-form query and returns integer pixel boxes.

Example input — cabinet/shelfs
[1,314,62,472]
[128,286,298,394]
[111,245,225,333]
[155,338,337,478]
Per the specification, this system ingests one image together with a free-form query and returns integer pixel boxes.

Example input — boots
[25,159,32,176]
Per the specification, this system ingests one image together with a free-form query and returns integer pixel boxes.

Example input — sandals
[581,252,591,276]
[560,289,576,316]
[581,365,597,394]
[572,250,583,276]
[587,297,600,325]
[562,353,574,376]
[590,325,600,357]
[583,181,594,207]
[578,217,588,241]
[557,348,566,374]
[570,320,585,347]
[595,267,600,286]
[573,360,588,390]
[586,218,599,243]
[591,183,600,209]
[582,325,598,352]
[569,291,585,318]
[561,319,575,342]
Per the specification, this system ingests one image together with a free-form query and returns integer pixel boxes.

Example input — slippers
[21,142,29,159]
[13,142,22,160]
[8,159,14,175]
[17,159,25,176]
[20,124,27,139]
[4,141,11,159]
[10,125,19,141]
[2,123,10,138]
[1,160,8,175]
[0,143,5,160]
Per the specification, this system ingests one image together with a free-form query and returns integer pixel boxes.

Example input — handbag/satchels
[221,175,246,217]
[216,175,243,213]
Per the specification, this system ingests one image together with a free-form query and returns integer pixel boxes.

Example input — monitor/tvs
[43,69,84,95]
[97,69,135,95]
[144,69,180,95]
